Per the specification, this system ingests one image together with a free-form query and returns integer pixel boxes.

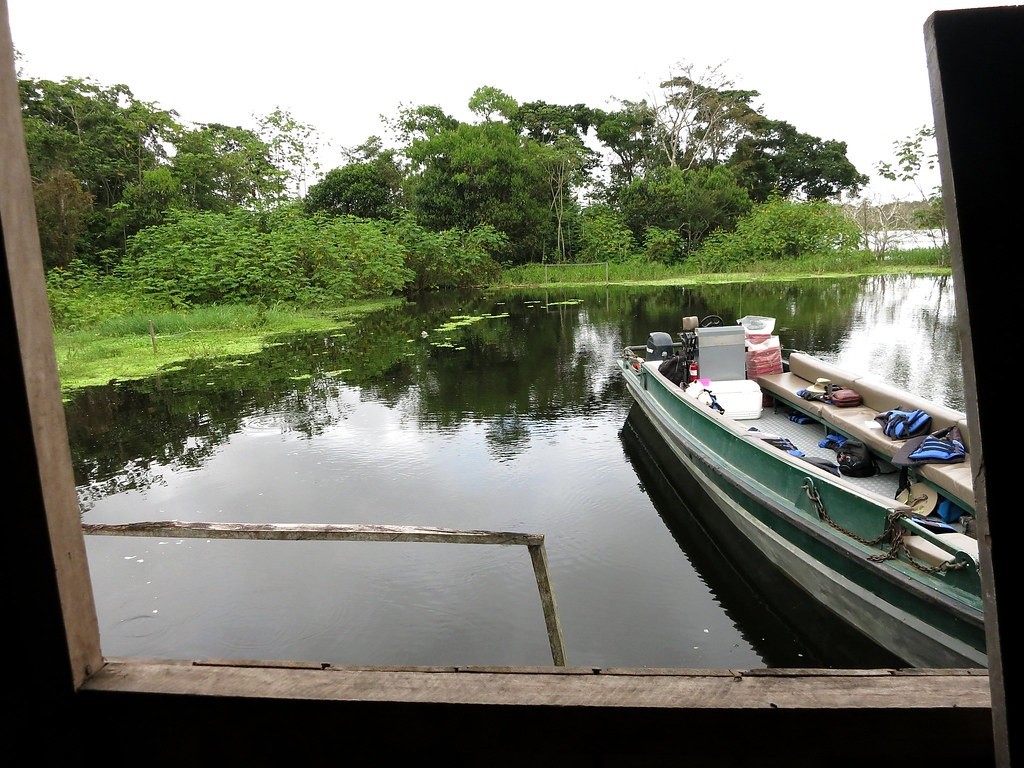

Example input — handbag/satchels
[823,386,862,407]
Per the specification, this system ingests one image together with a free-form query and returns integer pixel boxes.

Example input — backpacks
[837,439,881,478]
[658,350,691,392]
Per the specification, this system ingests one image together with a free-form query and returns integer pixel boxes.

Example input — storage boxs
[710,379,763,420]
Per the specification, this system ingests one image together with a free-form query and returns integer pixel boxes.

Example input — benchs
[639,351,979,572]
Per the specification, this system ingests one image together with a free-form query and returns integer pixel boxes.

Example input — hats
[894,482,938,517]
[806,377,832,394]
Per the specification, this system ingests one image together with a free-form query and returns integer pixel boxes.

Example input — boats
[617,314,991,672]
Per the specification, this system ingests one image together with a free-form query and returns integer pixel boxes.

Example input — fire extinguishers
[688,360,698,382]
